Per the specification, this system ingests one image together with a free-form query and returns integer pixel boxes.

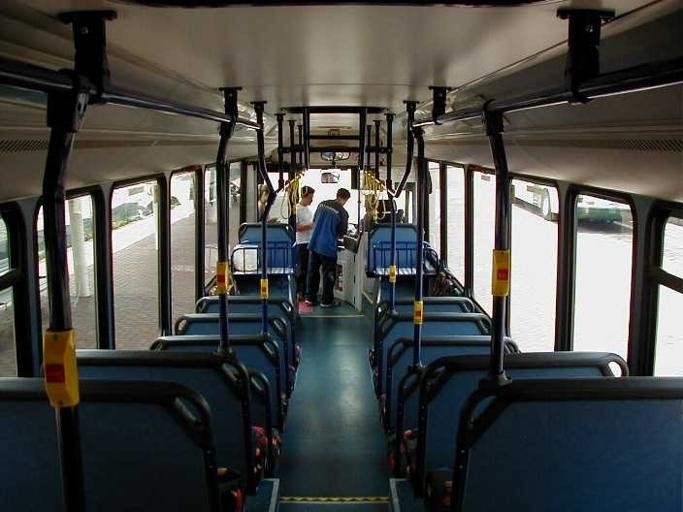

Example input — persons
[257,183,269,220]
[295,185,314,301]
[305,188,350,307]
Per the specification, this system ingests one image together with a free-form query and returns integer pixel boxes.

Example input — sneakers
[305,299,318,305]
[321,302,342,307]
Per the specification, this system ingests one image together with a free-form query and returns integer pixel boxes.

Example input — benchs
[1,291,300,512]
[234,218,435,289]
[367,295,682,508]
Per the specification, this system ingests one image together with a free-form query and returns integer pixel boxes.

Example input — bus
[510,179,628,222]
